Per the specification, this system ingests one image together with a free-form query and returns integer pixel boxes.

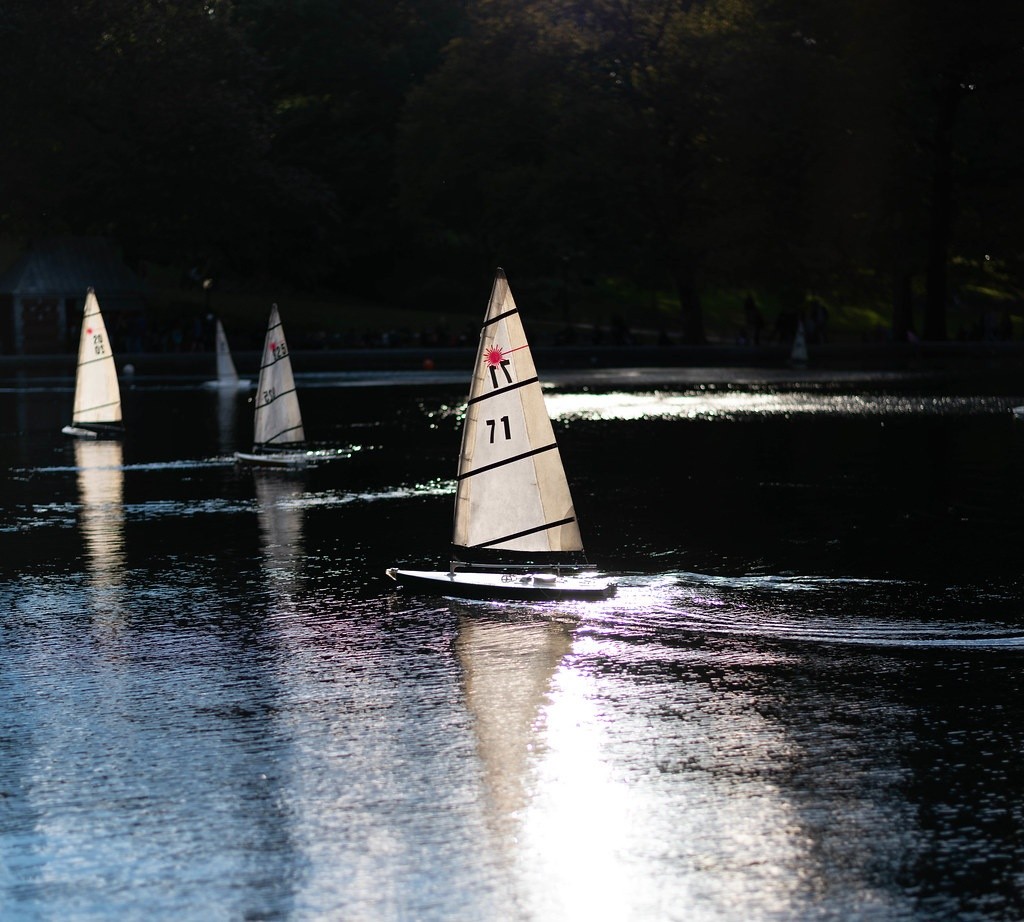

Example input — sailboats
[62,284,129,439]
[387,266,619,599]
[206,318,252,390]
[234,301,309,468]
